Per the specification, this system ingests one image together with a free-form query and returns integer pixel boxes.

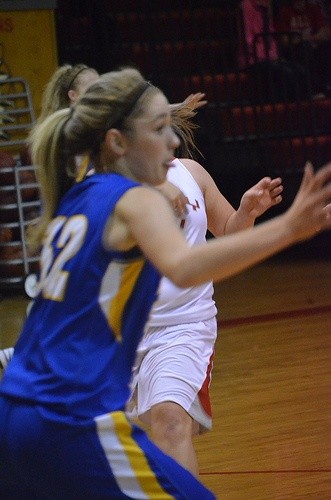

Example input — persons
[0,68,331,500]
[123,157,283,478]
[39,64,99,122]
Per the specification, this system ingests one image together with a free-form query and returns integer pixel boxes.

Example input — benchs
[58,0,331,181]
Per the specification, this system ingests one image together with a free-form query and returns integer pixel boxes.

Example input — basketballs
[0,149,41,277]
